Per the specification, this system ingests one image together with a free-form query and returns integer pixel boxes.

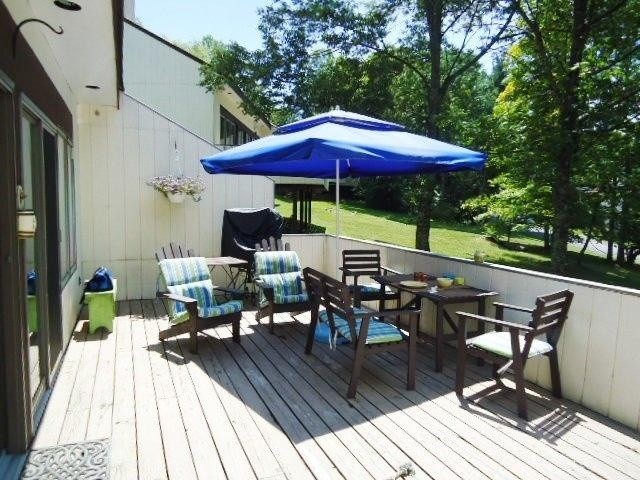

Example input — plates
[400,280,428,288]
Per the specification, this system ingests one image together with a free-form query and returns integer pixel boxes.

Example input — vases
[164,189,189,205]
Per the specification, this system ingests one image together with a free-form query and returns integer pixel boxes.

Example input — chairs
[217,203,285,307]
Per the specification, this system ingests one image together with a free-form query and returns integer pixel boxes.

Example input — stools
[83,278,119,336]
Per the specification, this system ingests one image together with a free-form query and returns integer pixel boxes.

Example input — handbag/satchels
[86,266,112,292]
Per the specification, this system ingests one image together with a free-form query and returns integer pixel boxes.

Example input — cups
[454,277,464,285]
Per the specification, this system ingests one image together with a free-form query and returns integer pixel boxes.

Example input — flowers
[141,171,211,207]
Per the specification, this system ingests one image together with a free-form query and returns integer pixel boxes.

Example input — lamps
[14,184,38,242]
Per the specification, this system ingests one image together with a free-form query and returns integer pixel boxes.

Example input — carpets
[15,432,112,480]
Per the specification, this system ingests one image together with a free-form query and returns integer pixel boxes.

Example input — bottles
[448,272,455,280]
[443,272,447,278]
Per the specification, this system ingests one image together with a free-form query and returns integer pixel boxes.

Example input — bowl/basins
[437,278,453,287]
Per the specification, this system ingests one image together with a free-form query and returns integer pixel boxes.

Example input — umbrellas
[199,105,488,283]
